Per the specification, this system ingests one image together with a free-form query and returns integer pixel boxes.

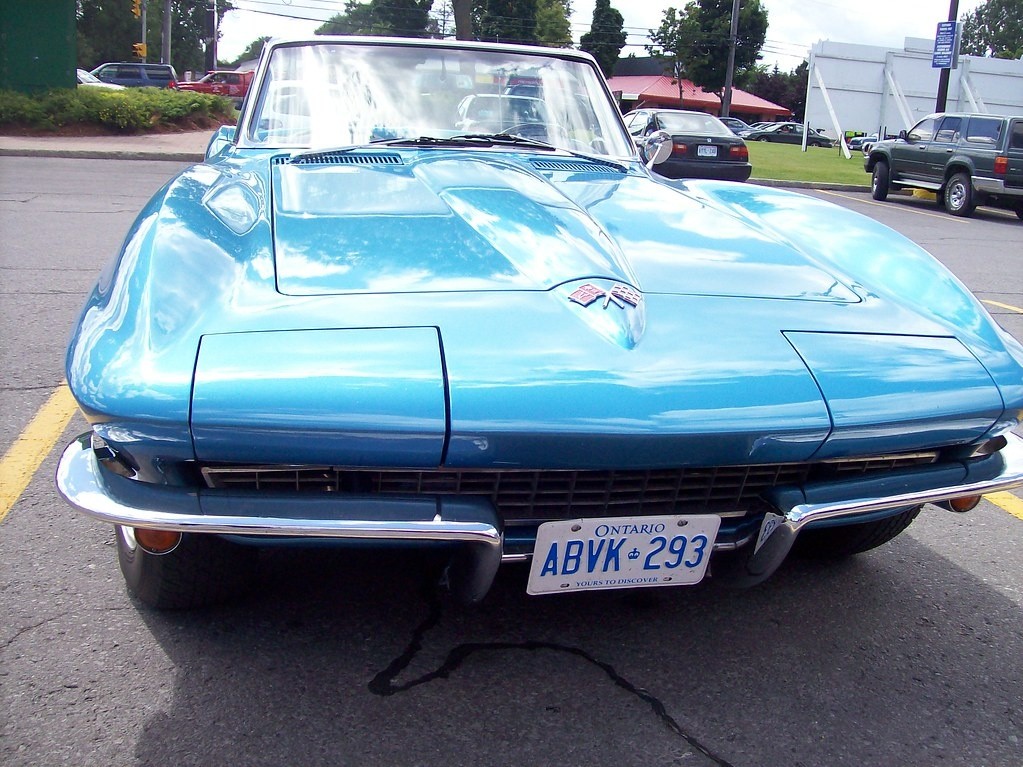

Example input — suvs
[89,62,178,89]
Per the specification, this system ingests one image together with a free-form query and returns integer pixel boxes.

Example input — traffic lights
[132,42,146,57]
[131,0,140,17]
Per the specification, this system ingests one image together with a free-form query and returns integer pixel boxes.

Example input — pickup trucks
[177,71,254,107]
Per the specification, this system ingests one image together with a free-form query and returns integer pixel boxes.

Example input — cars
[591,109,751,183]
[51,36,1023,616]
[739,122,832,148]
[75,68,124,91]
[848,132,879,153]
[749,122,766,128]
[864,113,1023,222]
[705,117,754,136]
